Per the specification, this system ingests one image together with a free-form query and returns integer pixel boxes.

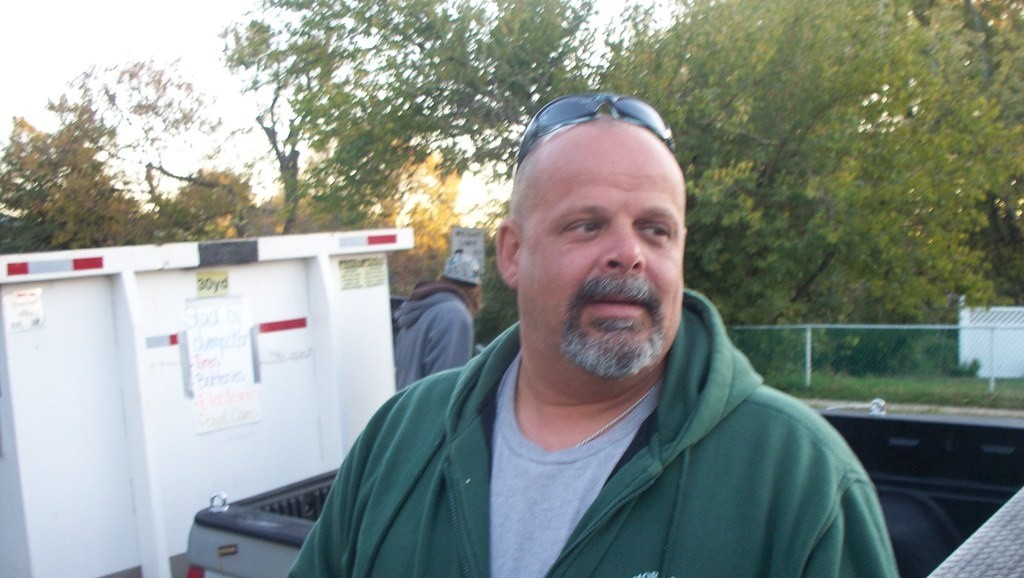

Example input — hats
[442,250,482,286]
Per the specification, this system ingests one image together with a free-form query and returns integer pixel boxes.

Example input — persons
[276,90,897,578]
[392,250,483,391]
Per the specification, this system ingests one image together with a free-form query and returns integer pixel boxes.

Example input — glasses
[518,92,678,168]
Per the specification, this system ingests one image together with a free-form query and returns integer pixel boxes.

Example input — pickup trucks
[189,395,1021,577]
